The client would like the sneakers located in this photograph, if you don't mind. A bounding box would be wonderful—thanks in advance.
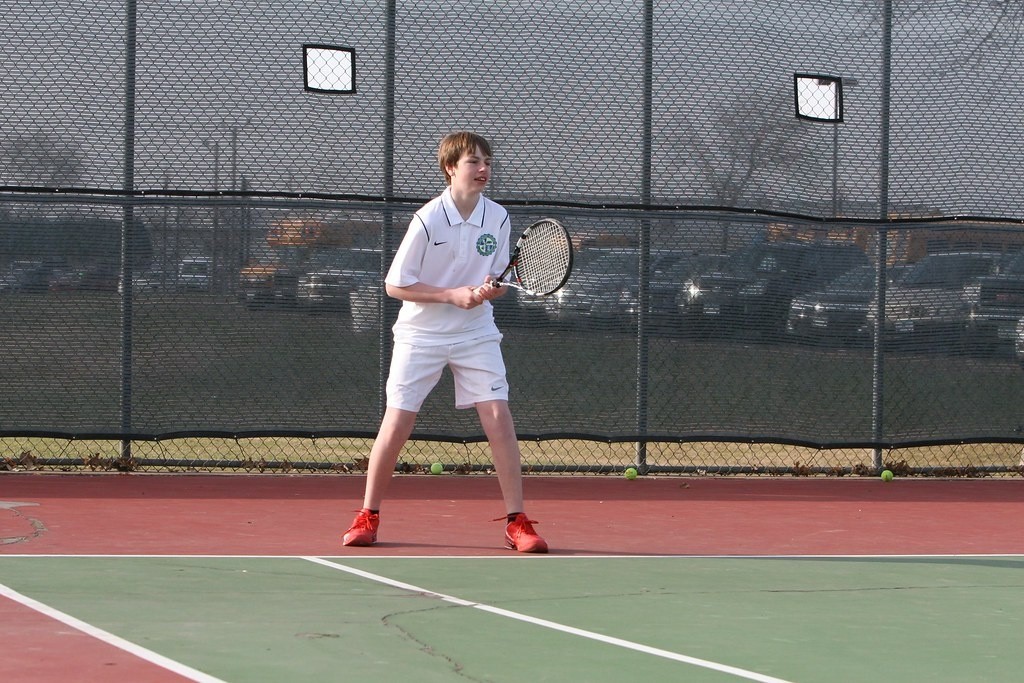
[488,513,548,553]
[340,509,380,546]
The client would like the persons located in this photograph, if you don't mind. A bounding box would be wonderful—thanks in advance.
[342,132,548,552]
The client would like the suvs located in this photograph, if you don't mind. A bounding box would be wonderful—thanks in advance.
[679,241,868,333]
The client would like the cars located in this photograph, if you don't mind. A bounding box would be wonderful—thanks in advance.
[508,248,703,318]
[968,252,1024,357]
[3,250,384,312]
[866,250,1002,343]
[787,262,910,336]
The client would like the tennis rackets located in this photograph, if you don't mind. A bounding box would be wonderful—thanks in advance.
[472,217,575,298]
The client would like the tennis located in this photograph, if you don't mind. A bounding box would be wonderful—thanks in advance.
[626,468,637,480]
[431,463,442,474]
[881,470,893,482]
